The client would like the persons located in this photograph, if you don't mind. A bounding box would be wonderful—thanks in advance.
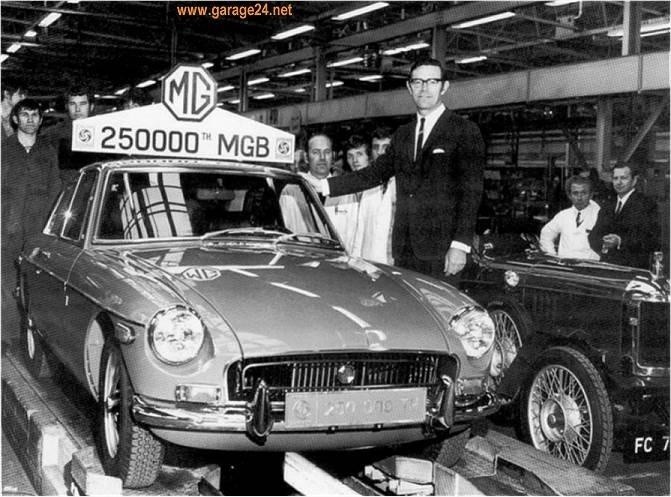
[350,126,400,268]
[39,84,106,193]
[297,55,487,295]
[338,132,373,202]
[1,69,30,144]
[1,96,62,357]
[538,171,604,262]
[588,160,663,270]
[280,131,358,255]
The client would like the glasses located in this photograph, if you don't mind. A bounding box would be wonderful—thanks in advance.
[410,78,441,88]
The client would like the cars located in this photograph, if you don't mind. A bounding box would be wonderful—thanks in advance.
[445,209,669,481]
[14,161,498,489]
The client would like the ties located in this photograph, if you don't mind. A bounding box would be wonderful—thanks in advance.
[576,212,581,225]
[615,201,622,215]
[416,119,424,158]
